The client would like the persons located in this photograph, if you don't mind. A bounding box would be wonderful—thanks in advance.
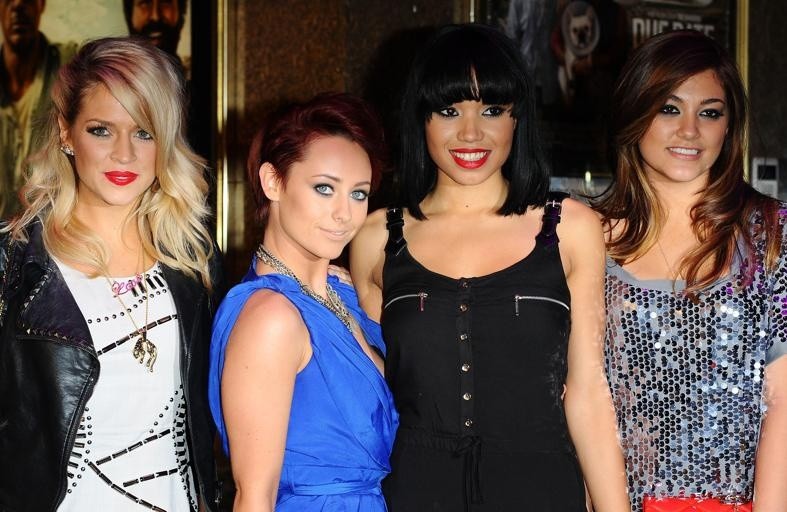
[0,35,236,512]
[207,105,399,512]
[588,29,787,512]
[349,22,632,512]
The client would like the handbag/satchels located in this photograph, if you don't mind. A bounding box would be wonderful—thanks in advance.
[642,490,753,512]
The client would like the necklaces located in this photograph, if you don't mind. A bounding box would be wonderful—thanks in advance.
[255,242,357,341]
[104,243,160,374]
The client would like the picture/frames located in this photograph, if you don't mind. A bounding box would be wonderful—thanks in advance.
[452,1,754,202]
[1,1,250,261]
